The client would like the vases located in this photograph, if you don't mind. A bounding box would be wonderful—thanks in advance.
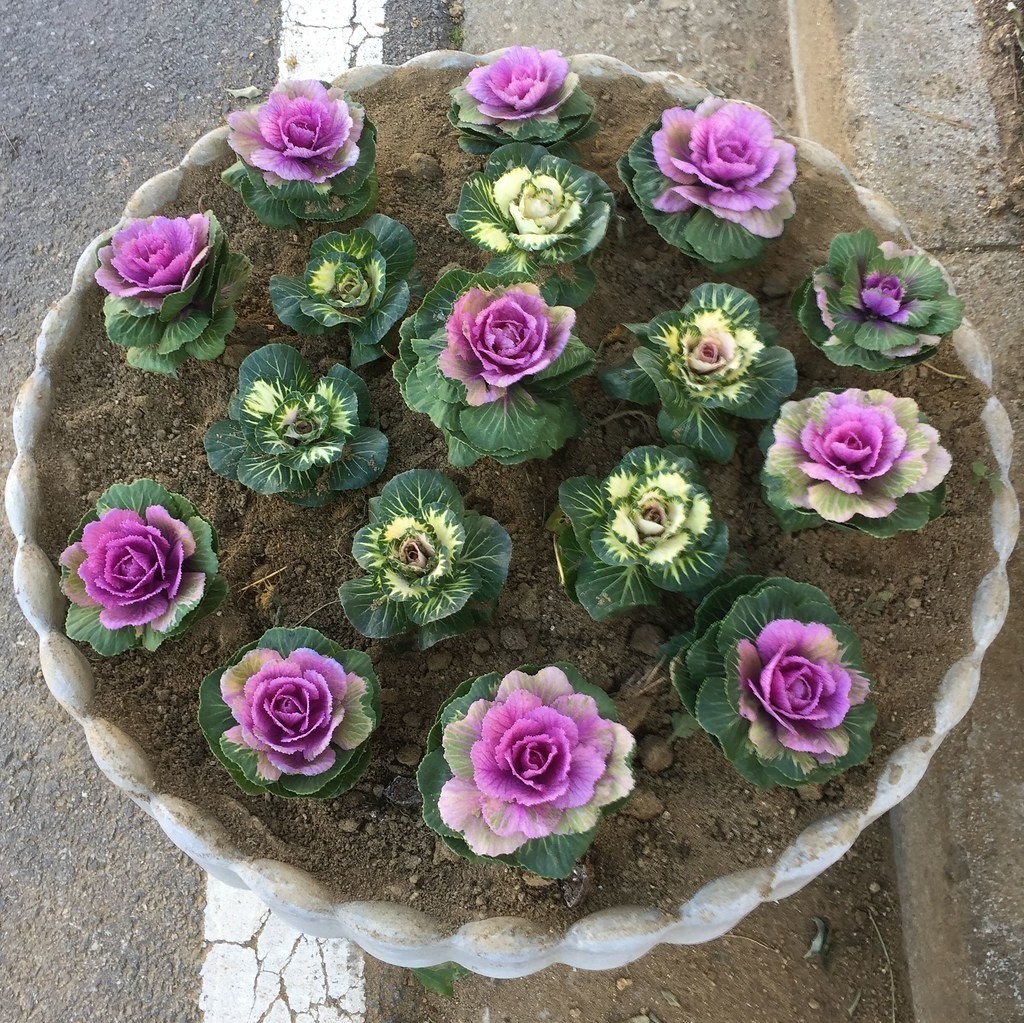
[4,49,1020,982]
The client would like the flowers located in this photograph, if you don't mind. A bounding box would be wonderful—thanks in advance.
[391,270,596,470]
[615,95,800,270]
[755,382,956,540]
[268,213,419,368]
[337,468,514,652]
[203,342,390,508]
[55,476,224,658]
[549,444,733,620]
[220,79,380,230]
[416,657,640,881]
[93,210,251,376]
[596,281,799,466]
[659,572,882,789]
[196,624,386,801]
[797,226,965,370]
[450,46,602,164]
[446,141,617,308]
